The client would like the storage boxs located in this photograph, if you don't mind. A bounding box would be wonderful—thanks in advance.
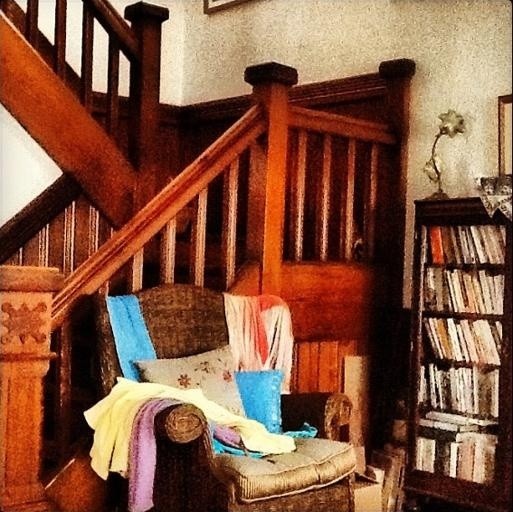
[351,471,383,512]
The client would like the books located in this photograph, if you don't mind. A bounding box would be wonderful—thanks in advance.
[412,223,507,487]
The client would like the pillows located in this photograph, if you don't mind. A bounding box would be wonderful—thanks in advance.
[132,344,247,418]
[235,364,286,432]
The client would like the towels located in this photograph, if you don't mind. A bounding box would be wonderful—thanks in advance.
[104,292,157,383]
[82,376,317,512]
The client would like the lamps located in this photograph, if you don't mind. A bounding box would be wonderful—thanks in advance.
[423,110,466,198]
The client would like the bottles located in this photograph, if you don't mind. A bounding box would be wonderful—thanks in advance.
[391,400,409,446]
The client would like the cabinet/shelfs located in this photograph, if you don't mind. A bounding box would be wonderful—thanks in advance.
[400,196,512,512]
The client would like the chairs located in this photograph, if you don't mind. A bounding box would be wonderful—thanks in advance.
[94,283,356,512]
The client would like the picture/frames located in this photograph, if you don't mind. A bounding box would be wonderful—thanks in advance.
[497,94,512,179]
[202,0,250,13]
